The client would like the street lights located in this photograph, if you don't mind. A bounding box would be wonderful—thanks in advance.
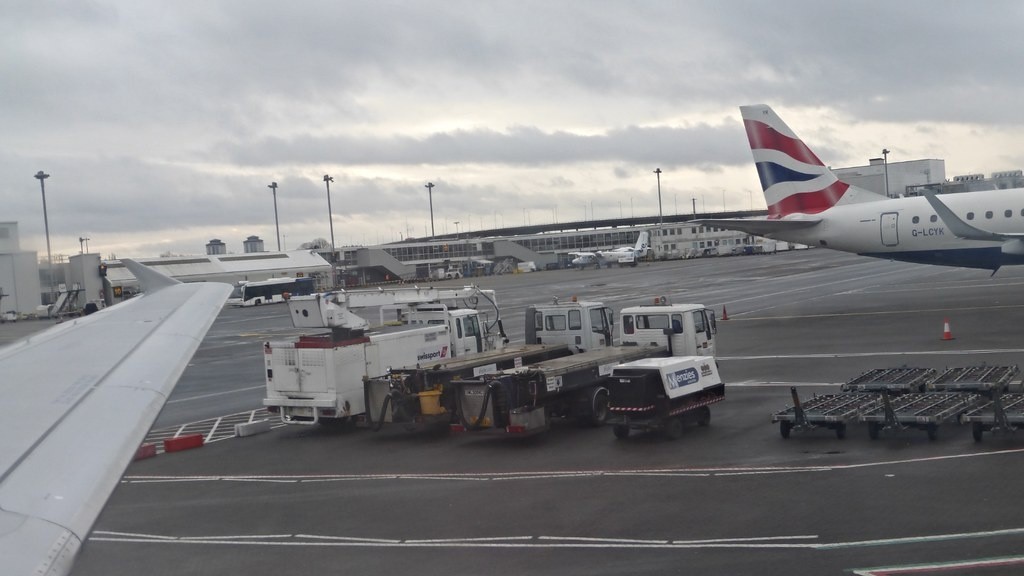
[881,148,890,197]
[454,221,460,238]
[653,168,664,242]
[268,181,283,252]
[323,174,335,263]
[34,170,57,304]
[425,181,435,239]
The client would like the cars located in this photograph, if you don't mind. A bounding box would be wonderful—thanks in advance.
[654,247,718,261]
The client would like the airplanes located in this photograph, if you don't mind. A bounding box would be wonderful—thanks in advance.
[686,104,1024,278]
[568,231,649,271]
[0,256,235,576]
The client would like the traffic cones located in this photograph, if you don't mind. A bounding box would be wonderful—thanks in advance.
[720,304,730,321]
[939,317,956,341]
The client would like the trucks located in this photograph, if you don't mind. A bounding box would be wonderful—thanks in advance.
[362,294,614,433]
[260,281,509,428]
[732,243,763,256]
[443,259,494,280]
[448,294,718,437]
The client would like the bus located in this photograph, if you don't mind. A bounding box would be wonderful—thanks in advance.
[240,276,318,307]
